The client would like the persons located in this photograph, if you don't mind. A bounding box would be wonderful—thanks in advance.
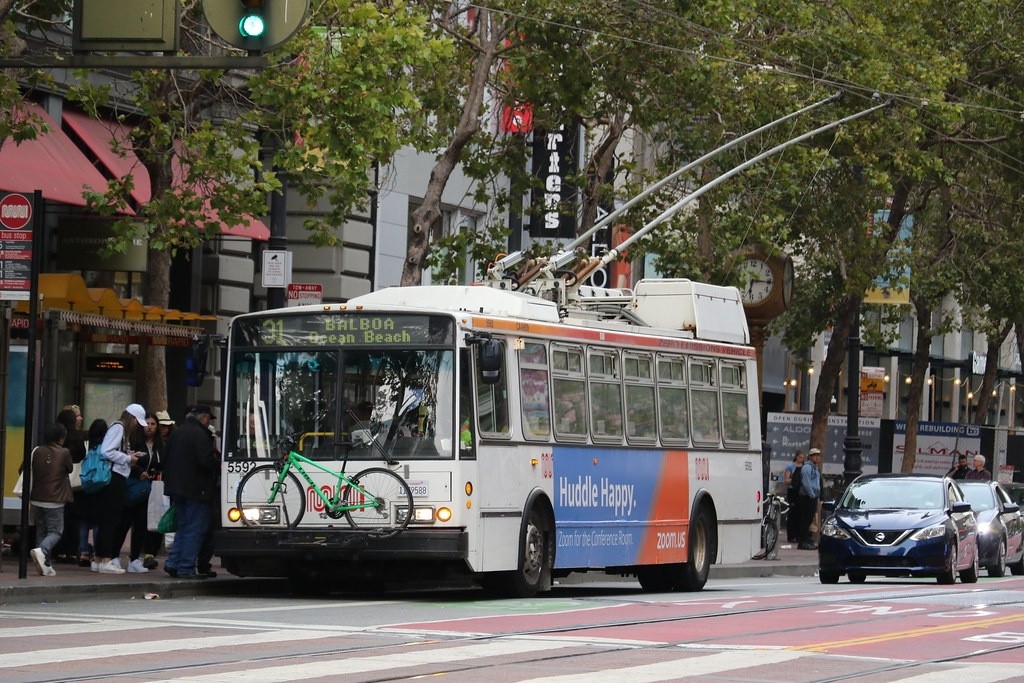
[964,455,991,481]
[797,448,820,550]
[785,450,805,544]
[945,455,971,479]
[416,399,508,454]
[18,404,221,579]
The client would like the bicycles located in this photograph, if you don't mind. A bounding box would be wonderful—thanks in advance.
[236,427,413,542]
[751,493,779,560]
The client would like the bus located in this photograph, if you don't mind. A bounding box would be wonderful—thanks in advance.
[220,89,894,598]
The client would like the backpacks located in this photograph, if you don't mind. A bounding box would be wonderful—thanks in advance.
[78,423,124,490]
[790,463,813,490]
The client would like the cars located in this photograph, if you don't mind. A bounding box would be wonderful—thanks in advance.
[817,472,980,585]
[954,480,1024,577]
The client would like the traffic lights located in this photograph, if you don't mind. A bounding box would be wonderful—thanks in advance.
[238,0,266,38]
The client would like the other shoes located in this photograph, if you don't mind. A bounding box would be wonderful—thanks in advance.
[78,552,94,567]
[788,539,797,543]
[199,568,217,577]
[127,559,149,573]
[44,565,56,576]
[29,547,46,576]
[112,558,121,568]
[143,556,159,569]
[90,561,118,571]
[97,562,126,574]
[177,571,207,579]
[163,566,180,579]
[797,543,816,551]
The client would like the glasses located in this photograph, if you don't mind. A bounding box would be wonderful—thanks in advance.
[69,405,77,411]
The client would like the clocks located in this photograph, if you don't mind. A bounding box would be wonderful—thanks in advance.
[784,261,792,303]
[734,258,773,302]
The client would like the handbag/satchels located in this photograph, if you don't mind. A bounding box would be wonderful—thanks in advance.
[146,475,170,531]
[12,446,38,499]
[156,502,176,533]
[68,441,89,488]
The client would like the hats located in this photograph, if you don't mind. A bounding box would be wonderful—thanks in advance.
[125,404,148,427]
[190,405,217,420]
[806,449,821,459]
[207,425,218,438]
[155,410,176,426]
